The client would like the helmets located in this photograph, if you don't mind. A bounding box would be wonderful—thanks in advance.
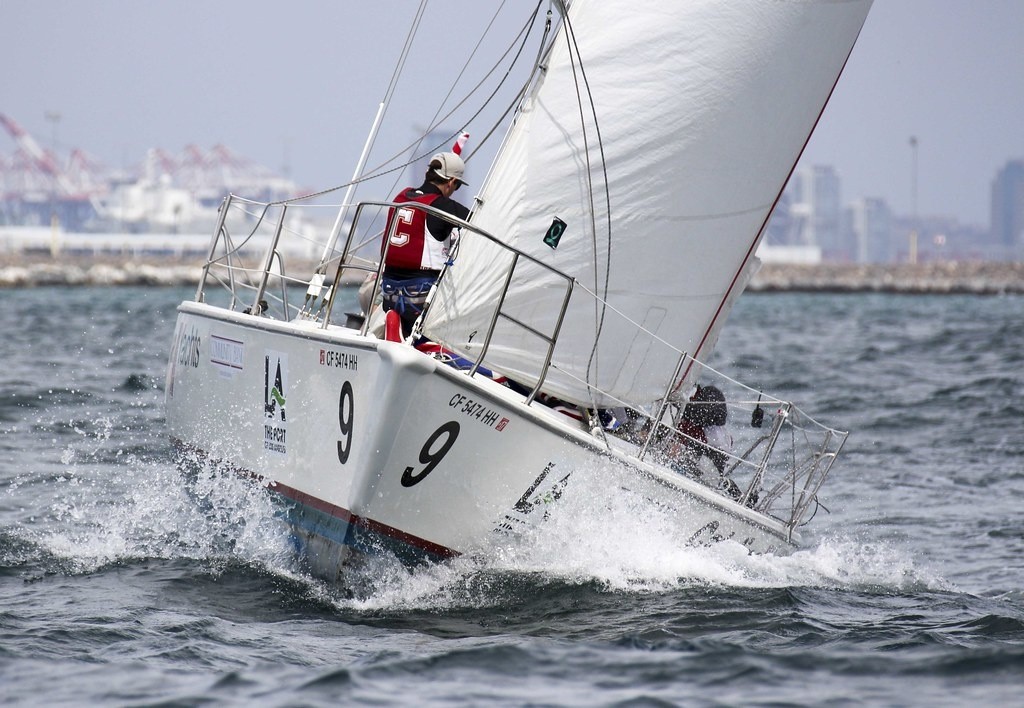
[684,384,727,427]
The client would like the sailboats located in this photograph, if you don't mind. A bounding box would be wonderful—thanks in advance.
[160,0,875,599]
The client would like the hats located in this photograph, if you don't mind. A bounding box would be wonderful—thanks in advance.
[429,152,470,186]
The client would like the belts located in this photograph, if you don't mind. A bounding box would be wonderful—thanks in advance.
[384,295,426,304]
[386,284,429,294]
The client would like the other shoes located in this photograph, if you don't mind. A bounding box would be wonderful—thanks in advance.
[384,309,405,343]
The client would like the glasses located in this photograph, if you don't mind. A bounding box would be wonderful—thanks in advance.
[455,180,462,190]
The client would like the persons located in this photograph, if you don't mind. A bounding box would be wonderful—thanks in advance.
[381,153,473,344]
